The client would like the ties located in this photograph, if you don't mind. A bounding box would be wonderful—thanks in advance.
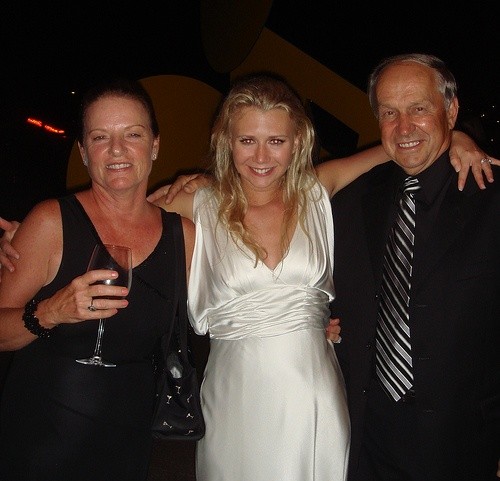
[375,176,422,402]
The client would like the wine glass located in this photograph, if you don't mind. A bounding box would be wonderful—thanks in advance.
[76,244,132,368]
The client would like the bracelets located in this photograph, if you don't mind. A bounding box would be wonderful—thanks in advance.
[23,296,64,343]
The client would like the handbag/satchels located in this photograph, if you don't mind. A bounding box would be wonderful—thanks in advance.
[151,212,210,441]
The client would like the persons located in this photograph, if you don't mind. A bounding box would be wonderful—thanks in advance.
[0,74,341,481]
[0,69,500,481]
[146,53,500,481]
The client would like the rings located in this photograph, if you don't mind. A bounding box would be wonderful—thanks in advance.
[480,158,492,164]
[88,299,97,311]
